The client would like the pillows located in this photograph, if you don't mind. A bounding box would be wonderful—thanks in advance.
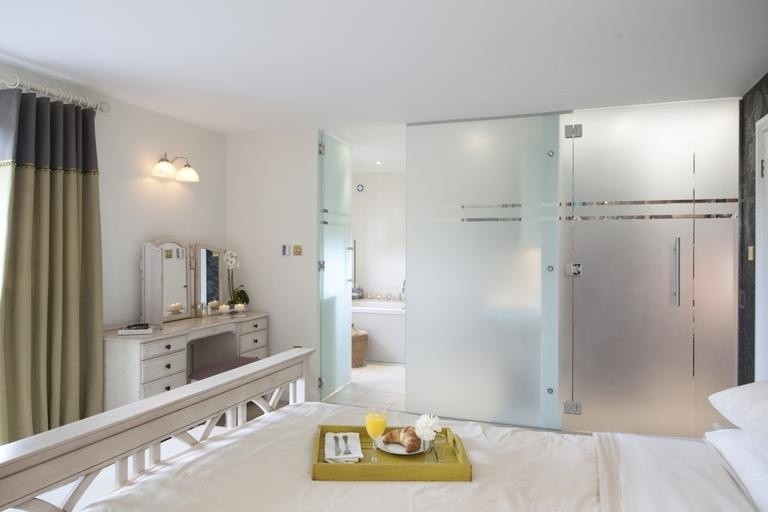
[701,366,766,509]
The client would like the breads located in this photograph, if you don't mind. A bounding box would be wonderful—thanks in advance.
[382,427,421,453]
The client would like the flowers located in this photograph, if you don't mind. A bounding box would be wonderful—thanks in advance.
[222,246,251,303]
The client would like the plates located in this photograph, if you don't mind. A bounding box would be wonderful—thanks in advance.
[375,432,429,455]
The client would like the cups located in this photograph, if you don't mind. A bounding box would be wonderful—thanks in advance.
[433,433,446,463]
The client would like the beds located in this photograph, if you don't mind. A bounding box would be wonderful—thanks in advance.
[0,345,767,511]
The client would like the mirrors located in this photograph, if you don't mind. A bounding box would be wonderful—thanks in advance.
[140,236,220,324]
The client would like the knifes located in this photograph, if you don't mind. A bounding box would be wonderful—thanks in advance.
[334,436,341,456]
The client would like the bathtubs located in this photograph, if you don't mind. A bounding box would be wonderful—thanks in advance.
[351,297,405,364]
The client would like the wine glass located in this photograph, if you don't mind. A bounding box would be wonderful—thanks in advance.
[365,408,386,463]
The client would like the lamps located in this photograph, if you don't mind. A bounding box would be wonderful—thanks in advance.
[151,151,199,182]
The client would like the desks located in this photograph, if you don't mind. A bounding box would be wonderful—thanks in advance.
[102,311,267,410]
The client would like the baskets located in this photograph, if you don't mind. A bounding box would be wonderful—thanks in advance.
[352,329,367,369]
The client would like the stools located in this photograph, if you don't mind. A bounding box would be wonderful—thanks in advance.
[189,356,260,382]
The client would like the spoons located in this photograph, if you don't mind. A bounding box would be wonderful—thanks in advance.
[343,436,351,454]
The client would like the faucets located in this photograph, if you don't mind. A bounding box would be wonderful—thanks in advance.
[400,277,405,295]
